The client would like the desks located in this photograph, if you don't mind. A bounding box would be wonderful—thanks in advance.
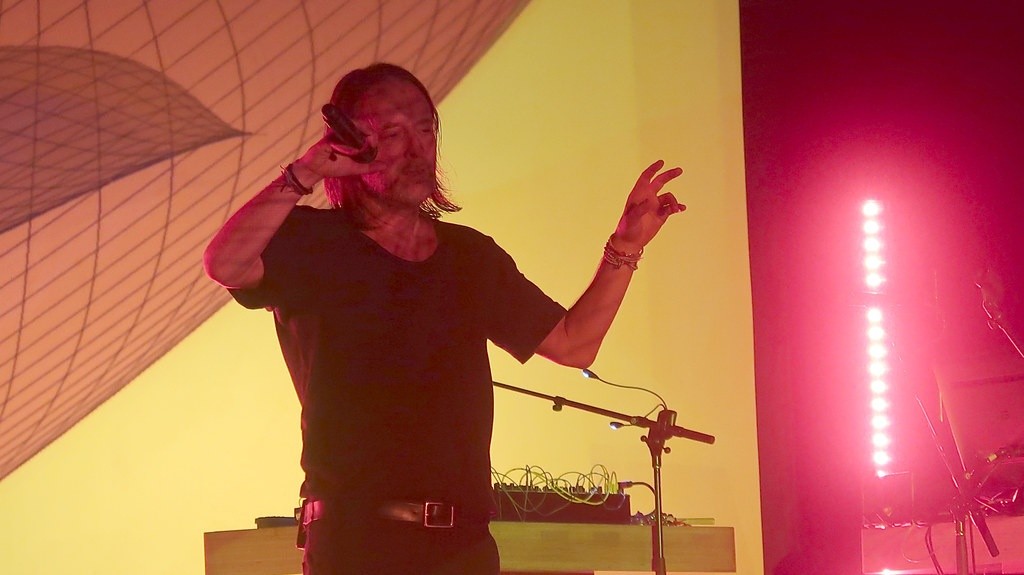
[204,520,734,575]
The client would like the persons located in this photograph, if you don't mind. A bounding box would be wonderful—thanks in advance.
[202,62,686,575]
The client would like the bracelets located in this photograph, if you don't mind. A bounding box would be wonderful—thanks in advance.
[604,234,643,270]
[280,162,313,196]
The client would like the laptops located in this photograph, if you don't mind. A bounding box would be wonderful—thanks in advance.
[862,472,914,528]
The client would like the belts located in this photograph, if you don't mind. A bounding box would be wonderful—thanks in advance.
[302,498,501,532]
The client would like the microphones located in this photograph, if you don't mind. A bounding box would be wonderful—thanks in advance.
[972,268,1002,321]
[984,438,1024,465]
[321,103,378,164]
